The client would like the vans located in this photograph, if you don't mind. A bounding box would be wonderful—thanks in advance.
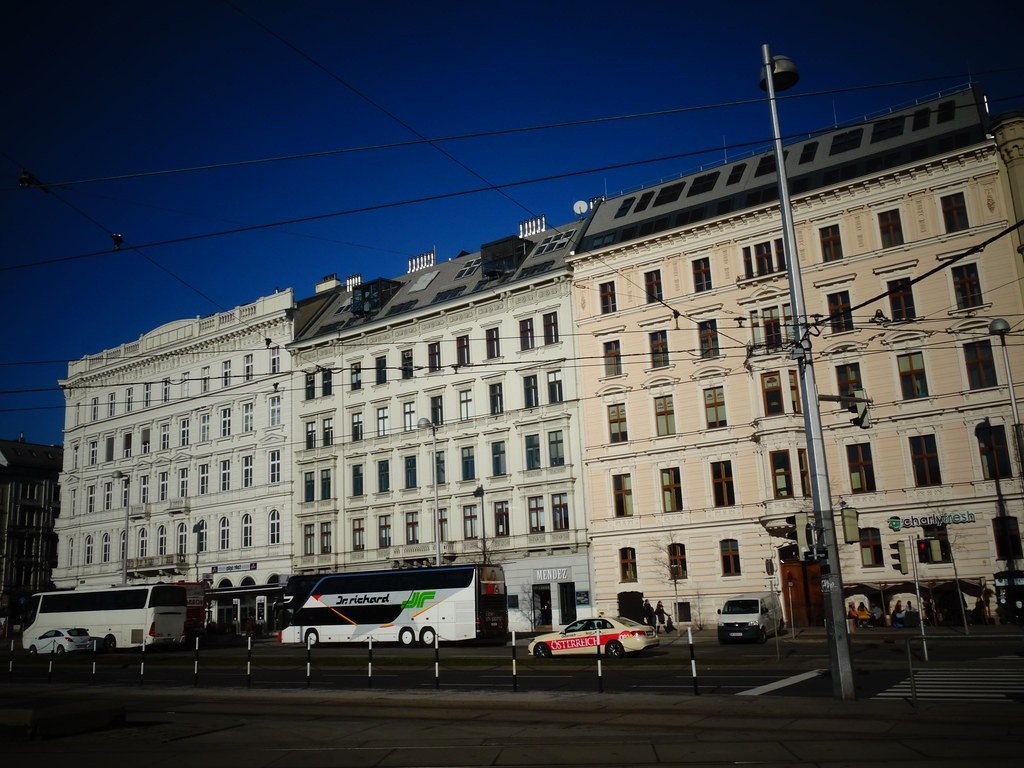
[717,592,786,645]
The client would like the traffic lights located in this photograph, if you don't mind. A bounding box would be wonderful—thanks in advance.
[785,513,811,563]
[847,391,871,429]
[889,540,909,575]
[916,539,930,563]
[940,538,951,561]
[840,509,862,545]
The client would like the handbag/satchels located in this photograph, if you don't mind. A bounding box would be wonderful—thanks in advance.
[655,609,661,616]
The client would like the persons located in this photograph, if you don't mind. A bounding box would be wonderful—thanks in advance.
[848,602,883,627]
[644,599,671,634]
[486,570,499,594]
[892,600,913,626]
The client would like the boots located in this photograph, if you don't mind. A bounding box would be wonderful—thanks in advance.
[663,626,668,633]
[657,626,662,634]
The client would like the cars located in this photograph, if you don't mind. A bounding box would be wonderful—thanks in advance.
[527,616,659,658]
[29,627,94,657]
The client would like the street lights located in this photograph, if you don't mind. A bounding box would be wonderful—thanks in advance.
[416,417,441,565]
[112,471,130,585]
[986,318,1024,474]
[756,43,854,699]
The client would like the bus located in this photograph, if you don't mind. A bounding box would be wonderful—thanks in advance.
[21,582,188,654]
[278,560,509,649]
[157,580,207,630]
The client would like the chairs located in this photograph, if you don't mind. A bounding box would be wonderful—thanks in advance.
[591,622,602,629]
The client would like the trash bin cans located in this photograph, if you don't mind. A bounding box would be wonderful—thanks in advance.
[886,614,891,626]
[848,619,857,634]
